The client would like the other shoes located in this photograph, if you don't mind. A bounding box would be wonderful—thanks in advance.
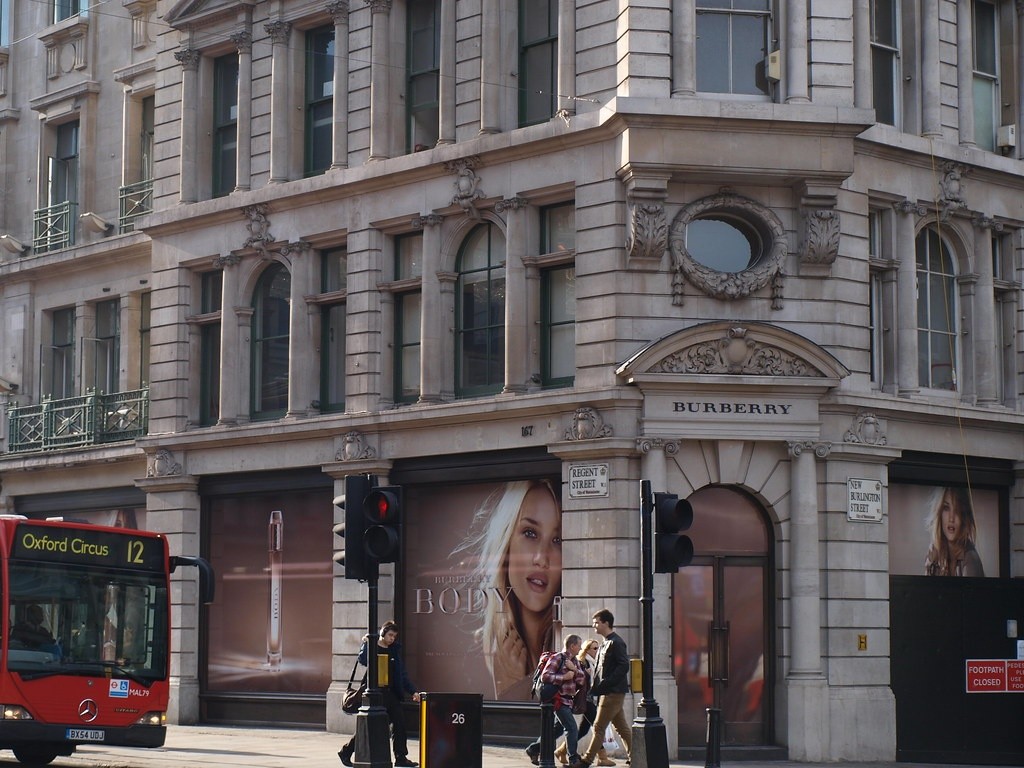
[338,750,353,767]
[563,761,589,768]
[569,758,587,768]
[525,748,539,765]
[395,757,419,767]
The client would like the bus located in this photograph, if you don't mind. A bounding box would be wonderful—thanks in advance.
[0,513,217,768]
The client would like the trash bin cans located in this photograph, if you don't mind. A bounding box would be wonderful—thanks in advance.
[419,691,483,768]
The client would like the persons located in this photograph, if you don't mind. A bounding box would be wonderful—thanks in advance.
[14,605,56,649]
[924,487,986,577]
[480,479,562,700]
[553,639,616,766]
[526,634,589,768]
[563,609,631,768]
[338,621,420,767]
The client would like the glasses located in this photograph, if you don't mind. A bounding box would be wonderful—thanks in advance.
[589,647,598,650]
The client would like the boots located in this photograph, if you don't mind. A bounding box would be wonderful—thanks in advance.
[597,749,616,766]
[554,741,568,764]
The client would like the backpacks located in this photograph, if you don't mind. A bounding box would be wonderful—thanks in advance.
[532,651,579,699]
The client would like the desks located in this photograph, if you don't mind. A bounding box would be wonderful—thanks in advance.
[107,409,138,427]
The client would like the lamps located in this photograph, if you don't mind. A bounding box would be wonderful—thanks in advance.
[0,234,31,254]
[79,212,114,233]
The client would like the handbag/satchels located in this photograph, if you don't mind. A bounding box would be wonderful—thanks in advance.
[590,722,619,750]
[531,674,559,704]
[571,683,586,715]
[341,683,367,714]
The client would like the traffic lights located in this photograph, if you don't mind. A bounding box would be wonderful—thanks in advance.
[653,491,694,574]
[360,486,402,563]
[332,473,380,581]
